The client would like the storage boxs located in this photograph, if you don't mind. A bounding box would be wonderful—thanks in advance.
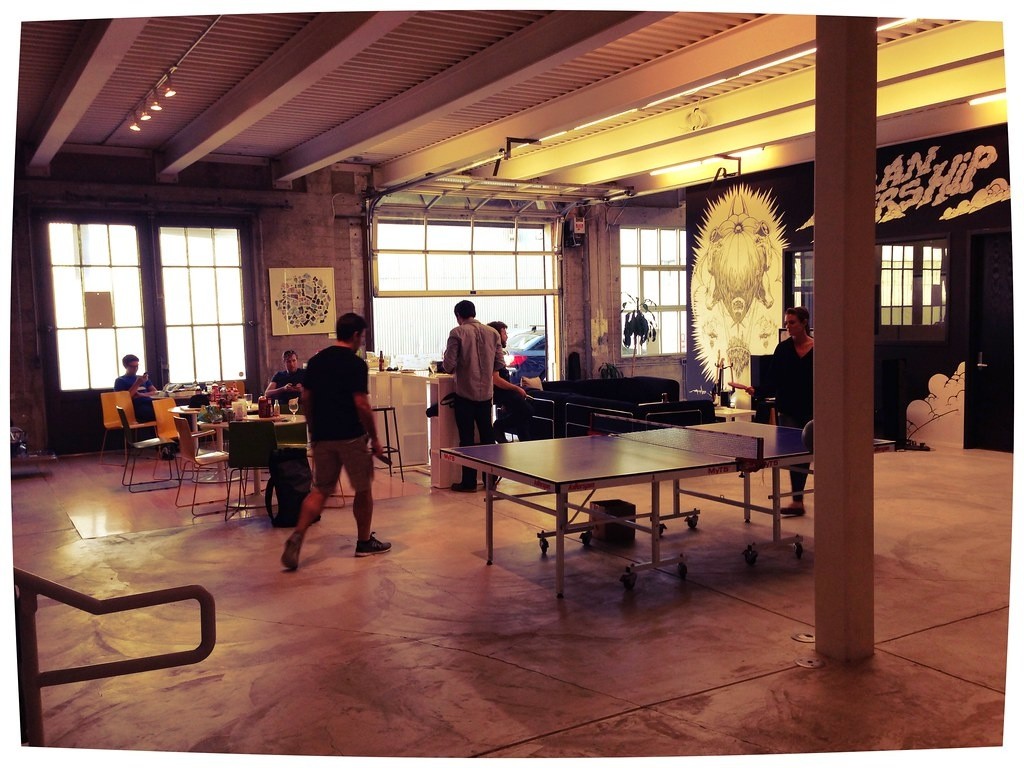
[589,499,636,541]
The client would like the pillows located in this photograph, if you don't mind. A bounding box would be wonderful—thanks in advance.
[520,376,543,390]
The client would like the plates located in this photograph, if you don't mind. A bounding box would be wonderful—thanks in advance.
[399,369,415,373]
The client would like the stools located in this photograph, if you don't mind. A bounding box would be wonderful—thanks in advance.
[372,404,406,483]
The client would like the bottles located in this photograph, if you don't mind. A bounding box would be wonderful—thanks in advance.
[196,381,247,423]
[258,392,280,418]
[379,351,384,371]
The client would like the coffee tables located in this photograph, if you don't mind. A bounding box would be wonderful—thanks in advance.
[715,405,757,422]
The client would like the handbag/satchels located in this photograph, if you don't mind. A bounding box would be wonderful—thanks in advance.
[265,448,322,527]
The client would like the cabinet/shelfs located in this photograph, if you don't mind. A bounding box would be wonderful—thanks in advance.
[429,381,441,488]
[396,378,429,468]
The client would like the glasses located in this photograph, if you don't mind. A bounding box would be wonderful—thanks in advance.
[127,365,138,369]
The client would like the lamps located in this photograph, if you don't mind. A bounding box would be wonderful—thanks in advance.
[129,73,177,132]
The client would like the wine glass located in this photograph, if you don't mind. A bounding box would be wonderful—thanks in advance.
[429,359,437,377]
[288,399,298,419]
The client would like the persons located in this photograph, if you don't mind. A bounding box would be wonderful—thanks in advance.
[265,350,306,412]
[114,354,175,459]
[444,301,505,492]
[486,321,531,443]
[282,312,392,569]
[745,307,814,514]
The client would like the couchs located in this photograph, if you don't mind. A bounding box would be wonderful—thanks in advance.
[496,376,715,441]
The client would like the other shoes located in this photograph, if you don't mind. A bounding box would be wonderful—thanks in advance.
[781,507,805,515]
[484,482,496,491]
[451,482,476,493]
[162,452,174,460]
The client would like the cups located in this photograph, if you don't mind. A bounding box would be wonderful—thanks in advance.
[243,394,252,410]
[231,402,244,422]
[238,398,247,416]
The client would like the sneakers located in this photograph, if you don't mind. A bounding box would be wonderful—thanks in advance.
[355,531,392,555]
[282,532,303,570]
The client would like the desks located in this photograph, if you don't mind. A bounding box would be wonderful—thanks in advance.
[439,413,897,602]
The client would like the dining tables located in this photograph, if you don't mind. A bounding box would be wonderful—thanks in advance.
[167,403,260,483]
[144,388,214,460]
[195,414,310,509]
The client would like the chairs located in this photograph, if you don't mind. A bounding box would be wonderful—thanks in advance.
[173,415,235,517]
[115,405,182,494]
[97,390,158,468]
[225,420,283,522]
[217,380,246,397]
[152,396,196,481]
